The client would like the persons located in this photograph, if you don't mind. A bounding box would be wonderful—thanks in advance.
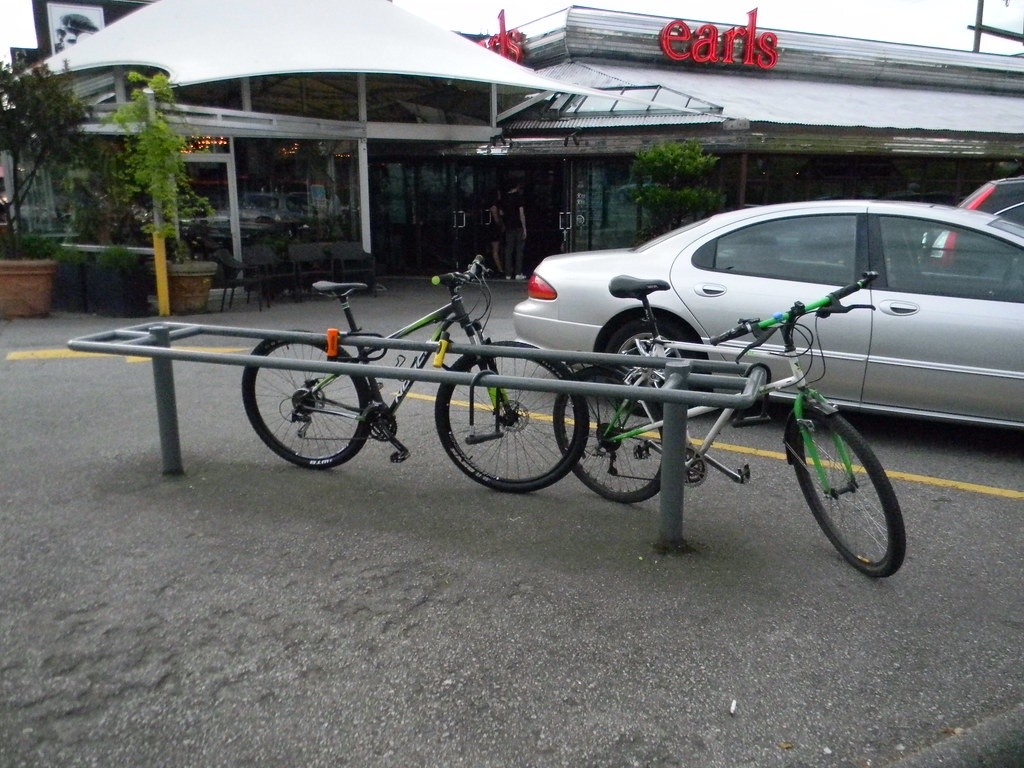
[483,188,505,278]
[498,176,527,279]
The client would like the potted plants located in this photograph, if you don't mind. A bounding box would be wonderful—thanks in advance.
[98,70,217,315]
[0,50,137,319]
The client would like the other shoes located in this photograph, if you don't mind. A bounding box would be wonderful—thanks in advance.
[505,276,512,280]
[515,273,527,281]
[498,271,504,276]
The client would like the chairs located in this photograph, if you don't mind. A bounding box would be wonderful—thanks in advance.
[216,248,271,311]
[242,240,378,302]
[889,234,914,286]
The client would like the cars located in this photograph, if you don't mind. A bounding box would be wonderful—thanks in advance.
[344,237,346,240]
[512,199,1024,431]
[239,180,347,240]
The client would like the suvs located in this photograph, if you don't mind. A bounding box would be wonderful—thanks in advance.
[921,177,1024,263]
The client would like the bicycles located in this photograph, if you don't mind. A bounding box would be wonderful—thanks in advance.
[552,272,910,578]
[239,254,590,493]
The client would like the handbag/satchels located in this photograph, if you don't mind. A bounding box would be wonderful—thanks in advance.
[515,238,534,268]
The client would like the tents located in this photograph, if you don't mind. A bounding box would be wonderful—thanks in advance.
[0,0,737,123]
